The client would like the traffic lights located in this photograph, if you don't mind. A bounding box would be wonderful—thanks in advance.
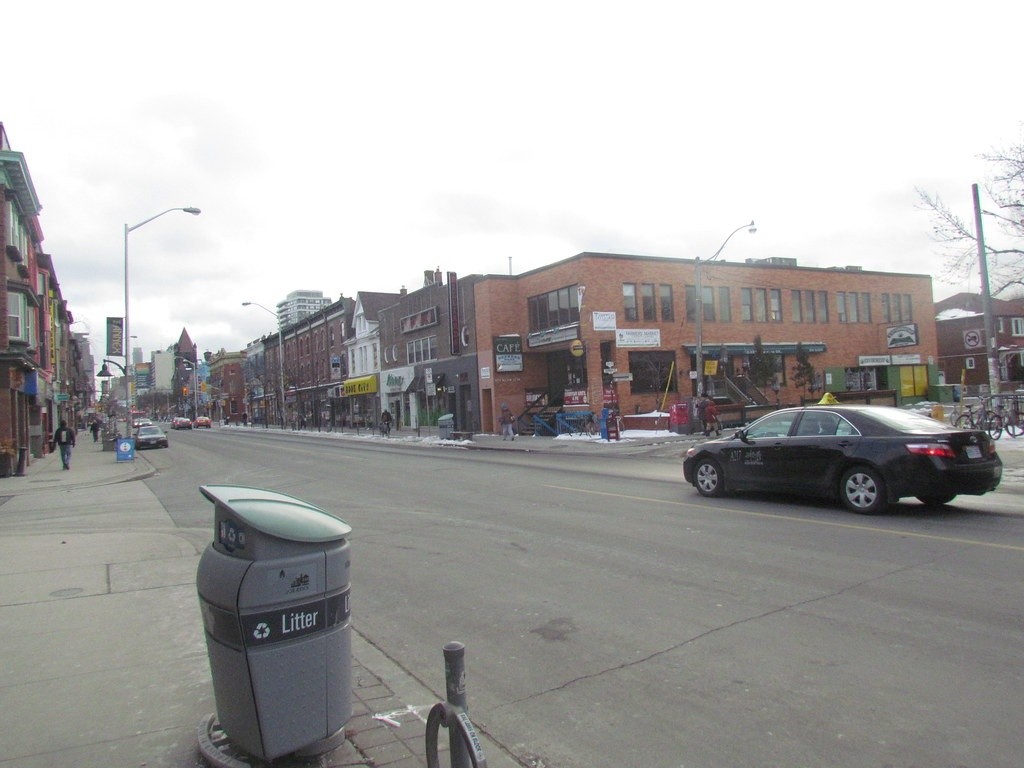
[184,388,188,396]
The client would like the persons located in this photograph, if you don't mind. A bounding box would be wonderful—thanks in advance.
[381,409,391,436]
[54,420,75,470]
[695,392,722,436]
[242,413,247,425]
[90,420,99,442]
[498,403,516,441]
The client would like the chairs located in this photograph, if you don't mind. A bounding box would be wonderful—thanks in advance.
[803,418,836,435]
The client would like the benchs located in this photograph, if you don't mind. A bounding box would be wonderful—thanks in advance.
[450,432,474,441]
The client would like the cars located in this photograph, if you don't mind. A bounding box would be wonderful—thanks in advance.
[132,426,169,450]
[194,417,211,428]
[134,419,152,428]
[683,405,1003,515]
[170,418,192,430]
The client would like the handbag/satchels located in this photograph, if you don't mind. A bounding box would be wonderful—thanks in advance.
[510,411,515,422]
[711,415,717,422]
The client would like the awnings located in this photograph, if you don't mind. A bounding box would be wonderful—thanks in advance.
[344,375,377,396]
[686,344,827,356]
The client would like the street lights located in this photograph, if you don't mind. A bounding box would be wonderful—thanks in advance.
[694,220,757,396]
[242,302,286,430]
[183,359,198,417]
[94,207,201,438]
[108,336,138,390]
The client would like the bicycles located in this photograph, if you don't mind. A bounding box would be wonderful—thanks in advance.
[988,394,1024,440]
[951,396,999,437]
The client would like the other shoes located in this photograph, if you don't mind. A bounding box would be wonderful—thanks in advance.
[706,430,710,436]
[716,431,721,436]
[702,432,706,435]
[63,463,69,470]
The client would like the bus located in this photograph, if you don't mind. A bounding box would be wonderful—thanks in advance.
[128,410,145,424]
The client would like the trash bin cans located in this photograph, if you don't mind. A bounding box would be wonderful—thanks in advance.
[438,413,454,440]
[196,483,354,768]
